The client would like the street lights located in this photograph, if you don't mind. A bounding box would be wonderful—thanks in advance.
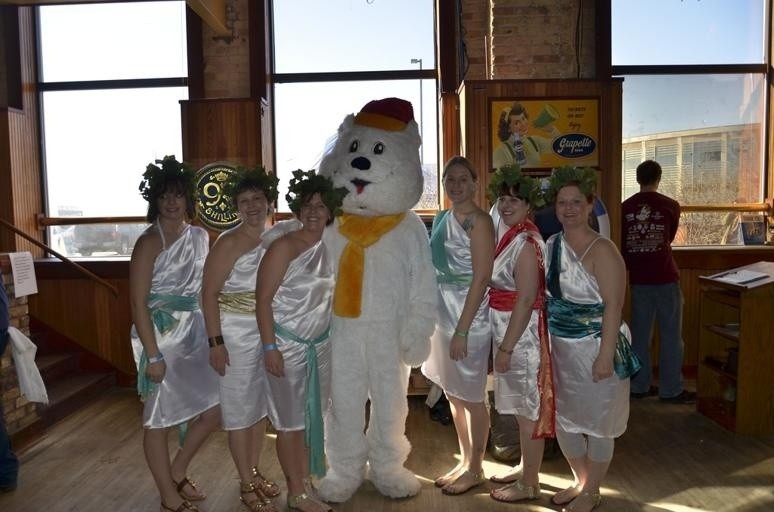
[411,58,424,166]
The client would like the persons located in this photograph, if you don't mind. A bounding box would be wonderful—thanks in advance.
[622,160,697,404]
[254,171,335,512]
[492,103,562,169]
[208,336,224,348]
[0,277,20,495]
[430,155,495,494]
[488,164,544,503]
[544,166,633,512]
[200,163,279,509]
[720,199,741,244]
[146,353,165,365]
[129,156,224,512]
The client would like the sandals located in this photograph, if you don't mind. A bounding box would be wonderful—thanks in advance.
[160,466,333,511]
[434,460,603,510]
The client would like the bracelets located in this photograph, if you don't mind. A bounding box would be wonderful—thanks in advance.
[496,345,513,355]
[262,344,278,351]
[454,328,469,337]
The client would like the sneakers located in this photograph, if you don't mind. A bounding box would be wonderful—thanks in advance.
[658,390,697,404]
[630,386,659,398]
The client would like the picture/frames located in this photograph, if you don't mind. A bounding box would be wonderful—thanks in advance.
[486,97,603,173]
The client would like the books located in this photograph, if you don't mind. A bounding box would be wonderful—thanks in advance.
[742,214,764,223]
[740,221,765,245]
[715,268,769,284]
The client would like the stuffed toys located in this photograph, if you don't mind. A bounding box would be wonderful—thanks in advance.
[260,97,437,504]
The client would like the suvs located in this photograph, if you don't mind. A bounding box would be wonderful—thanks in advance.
[74,223,145,256]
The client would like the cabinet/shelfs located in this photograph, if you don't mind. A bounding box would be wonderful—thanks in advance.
[696,262,773,447]
